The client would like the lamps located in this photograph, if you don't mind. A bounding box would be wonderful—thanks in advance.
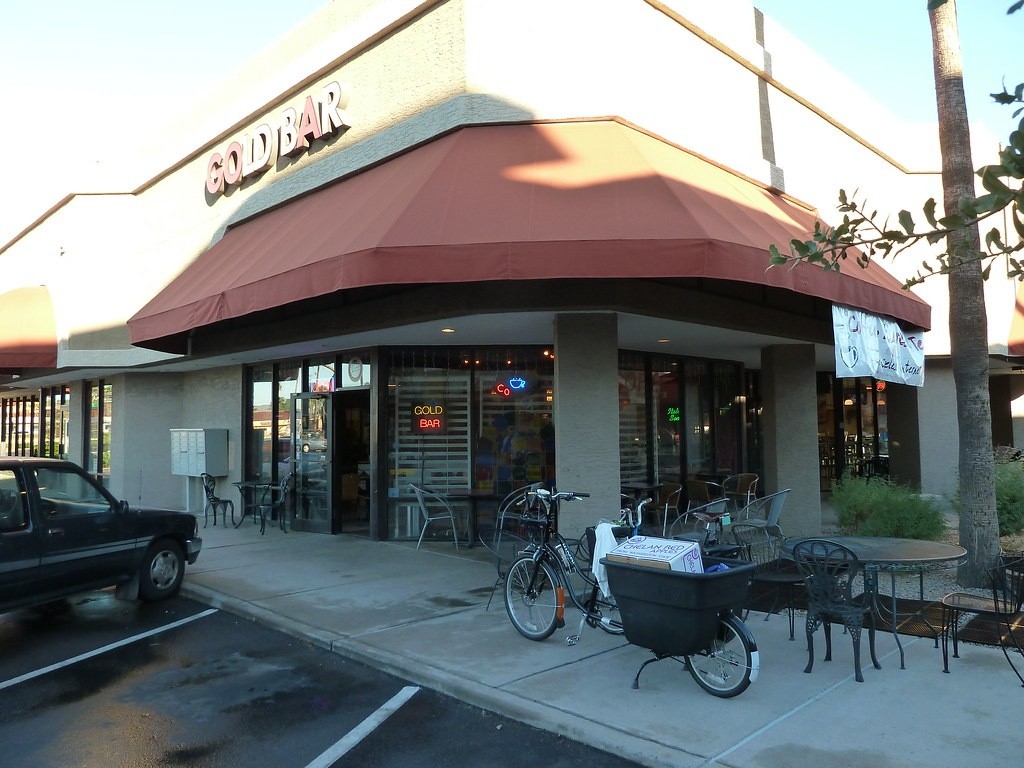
[844,379,853,405]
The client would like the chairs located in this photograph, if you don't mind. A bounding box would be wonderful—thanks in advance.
[476,528,558,611]
[258,472,296,536]
[491,481,547,553]
[649,471,1024,686]
[407,482,460,553]
[201,473,238,530]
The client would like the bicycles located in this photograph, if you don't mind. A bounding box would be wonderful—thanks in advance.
[503,488,760,698]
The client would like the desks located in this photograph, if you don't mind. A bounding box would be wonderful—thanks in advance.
[781,537,967,669]
[448,487,495,547]
[621,481,663,536]
[232,480,283,533]
[528,537,582,594]
[697,473,744,522]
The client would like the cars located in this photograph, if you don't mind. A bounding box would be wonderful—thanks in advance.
[262,436,327,489]
[0,457,202,615]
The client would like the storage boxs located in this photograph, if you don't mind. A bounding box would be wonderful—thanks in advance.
[606,534,704,573]
